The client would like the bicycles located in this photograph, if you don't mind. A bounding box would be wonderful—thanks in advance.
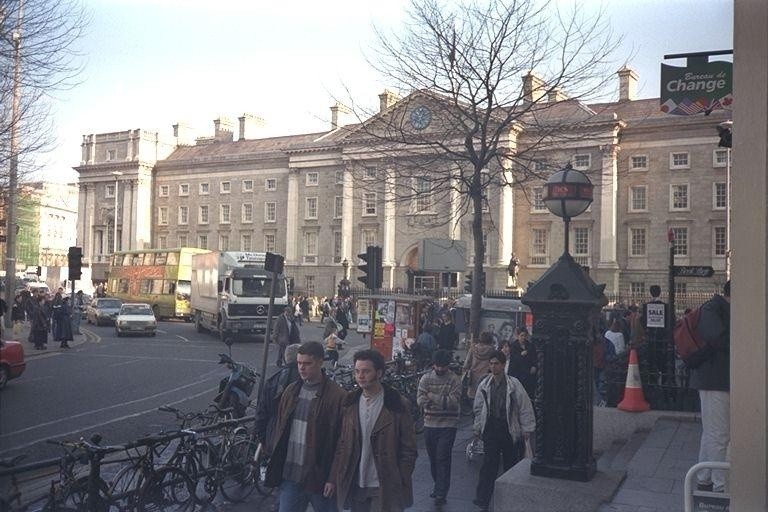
[322,350,475,434]
[0,404,275,512]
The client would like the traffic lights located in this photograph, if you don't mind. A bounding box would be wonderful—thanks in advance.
[69,247,83,280]
[464,271,485,295]
[357,246,383,289]
[37,266,41,276]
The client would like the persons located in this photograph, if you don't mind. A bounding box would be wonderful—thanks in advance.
[21,290,50,298]
[601,303,639,324]
[57,297,74,348]
[312,296,320,317]
[256,342,301,460]
[51,292,61,341]
[32,298,47,349]
[605,320,623,352]
[294,302,302,326]
[264,341,348,512]
[591,328,617,394]
[271,307,291,367]
[321,299,331,323]
[438,311,458,357]
[12,295,22,331]
[289,295,300,301]
[509,328,537,397]
[301,297,311,323]
[693,282,730,505]
[416,348,460,505]
[497,339,510,372]
[332,297,353,329]
[0,300,8,340]
[411,325,438,356]
[463,331,495,407]
[324,329,346,366]
[472,352,534,510]
[322,351,417,511]
[73,291,84,336]
[643,284,668,386]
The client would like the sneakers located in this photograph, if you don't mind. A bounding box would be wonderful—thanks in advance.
[435,495,447,507]
[473,499,489,512]
[431,488,436,497]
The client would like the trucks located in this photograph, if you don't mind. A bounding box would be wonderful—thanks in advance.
[190,251,294,342]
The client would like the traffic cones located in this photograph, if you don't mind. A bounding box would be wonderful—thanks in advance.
[614,349,652,413]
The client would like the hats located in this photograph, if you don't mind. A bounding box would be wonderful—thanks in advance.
[431,349,452,366]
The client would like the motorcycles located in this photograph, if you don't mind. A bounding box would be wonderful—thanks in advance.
[209,336,261,418]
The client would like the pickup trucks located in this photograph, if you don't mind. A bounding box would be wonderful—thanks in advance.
[114,303,157,336]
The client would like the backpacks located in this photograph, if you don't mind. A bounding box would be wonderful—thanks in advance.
[674,302,726,369]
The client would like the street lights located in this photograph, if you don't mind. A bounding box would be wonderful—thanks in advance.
[112,171,124,254]
[338,258,352,302]
[519,158,611,476]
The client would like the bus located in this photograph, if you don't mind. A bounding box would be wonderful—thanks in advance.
[104,249,213,322]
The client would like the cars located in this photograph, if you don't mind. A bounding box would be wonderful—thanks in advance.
[1,272,50,299]
[0,339,25,390]
[88,298,125,326]
